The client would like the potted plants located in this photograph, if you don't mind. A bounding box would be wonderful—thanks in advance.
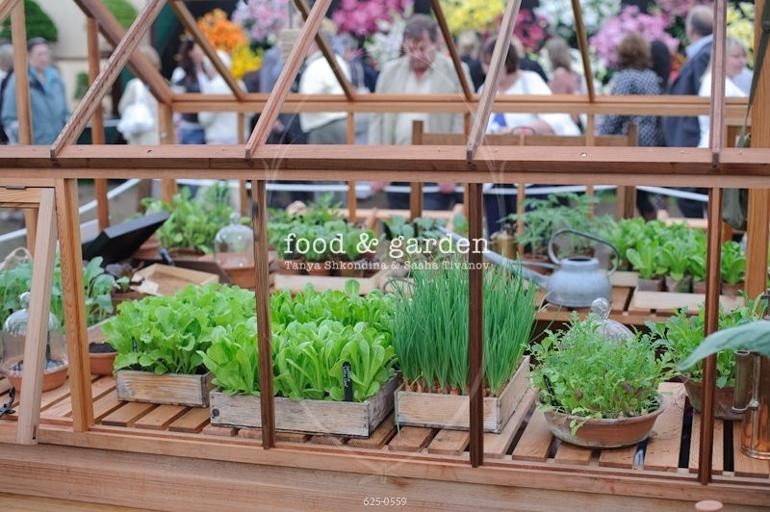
[0,178,769,462]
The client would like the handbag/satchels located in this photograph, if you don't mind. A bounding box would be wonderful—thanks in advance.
[721,131,748,229]
[117,100,155,136]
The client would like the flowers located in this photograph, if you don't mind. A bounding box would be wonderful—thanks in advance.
[178,2,757,96]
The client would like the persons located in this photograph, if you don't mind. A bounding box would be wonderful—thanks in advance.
[0,36,72,146]
[115,3,758,243]
[0,43,13,144]
